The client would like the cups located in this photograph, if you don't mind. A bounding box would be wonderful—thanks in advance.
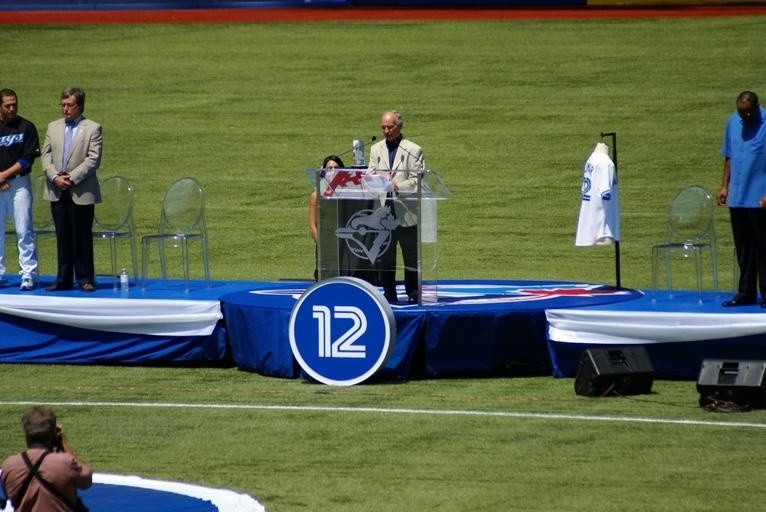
[353,140,365,165]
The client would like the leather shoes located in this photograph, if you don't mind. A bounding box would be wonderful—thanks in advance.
[44,280,75,292]
[721,291,766,309]
[78,280,95,292]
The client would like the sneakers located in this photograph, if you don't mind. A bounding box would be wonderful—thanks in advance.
[407,294,419,305]
[0,276,12,289]
[19,274,39,291]
[384,292,400,305]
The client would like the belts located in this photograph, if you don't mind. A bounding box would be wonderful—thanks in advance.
[4,170,29,182]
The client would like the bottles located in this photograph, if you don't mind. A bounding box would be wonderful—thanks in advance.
[120,267,129,292]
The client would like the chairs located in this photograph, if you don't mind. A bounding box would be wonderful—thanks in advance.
[91,175,143,291]
[651,184,721,306]
[5,176,56,290]
[733,248,761,299]
[140,175,210,294]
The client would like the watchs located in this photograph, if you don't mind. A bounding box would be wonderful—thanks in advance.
[66,176,72,182]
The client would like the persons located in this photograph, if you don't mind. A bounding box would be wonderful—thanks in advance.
[1,406,91,512]
[574,140,618,247]
[717,91,765,307]
[41,86,101,292]
[365,110,425,303]
[309,155,343,280]
[0,88,41,290]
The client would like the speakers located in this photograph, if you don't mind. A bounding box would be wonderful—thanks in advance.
[574,347,654,397]
[697,359,766,407]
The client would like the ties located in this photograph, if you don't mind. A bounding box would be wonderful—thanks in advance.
[388,144,396,171]
[61,121,76,174]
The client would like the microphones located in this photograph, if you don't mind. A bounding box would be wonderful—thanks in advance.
[334,136,376,157]
[394,140,423,164]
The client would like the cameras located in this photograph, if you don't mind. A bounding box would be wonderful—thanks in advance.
[55,428,62,449]
